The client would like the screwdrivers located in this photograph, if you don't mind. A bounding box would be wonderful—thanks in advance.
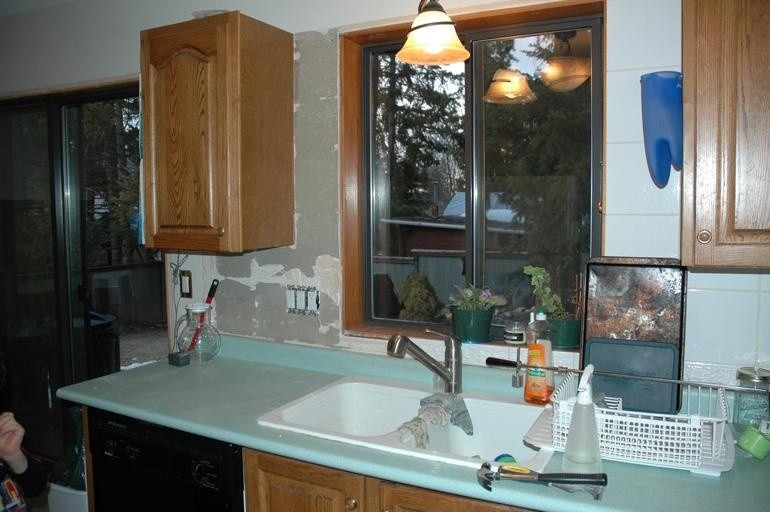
[497,464,604,498]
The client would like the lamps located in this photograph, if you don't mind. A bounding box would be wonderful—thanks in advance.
[395,1,469,67]
[482,29,591,108]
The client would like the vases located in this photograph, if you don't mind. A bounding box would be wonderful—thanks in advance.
[451,306,493,343]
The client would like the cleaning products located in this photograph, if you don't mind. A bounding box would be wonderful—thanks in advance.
[524,306,555,393]
[562,364,603,474]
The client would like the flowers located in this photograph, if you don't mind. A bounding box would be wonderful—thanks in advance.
[448,283,506,310]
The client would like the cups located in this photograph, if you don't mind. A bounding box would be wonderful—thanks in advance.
[504,319,526,346]
[737,427,770,461]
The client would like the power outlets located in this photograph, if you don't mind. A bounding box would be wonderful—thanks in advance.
[307,291,318,310]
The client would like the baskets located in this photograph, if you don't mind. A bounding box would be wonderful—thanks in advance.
[549,373,732,467]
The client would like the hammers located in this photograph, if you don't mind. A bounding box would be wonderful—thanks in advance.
[478,462,610,490]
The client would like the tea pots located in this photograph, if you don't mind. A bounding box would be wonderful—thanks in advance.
[176,303,220,360]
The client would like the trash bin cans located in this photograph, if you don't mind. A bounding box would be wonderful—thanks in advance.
[89,311,120,378]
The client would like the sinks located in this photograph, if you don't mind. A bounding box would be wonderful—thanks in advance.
[257,376,437,437]
[400,399,556,480]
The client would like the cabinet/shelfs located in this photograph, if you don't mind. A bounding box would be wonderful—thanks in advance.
[139,11,294,253]
[681,1,769,269]
[242,449,515,511]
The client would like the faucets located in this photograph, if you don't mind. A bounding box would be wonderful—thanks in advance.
[388,328,463,393]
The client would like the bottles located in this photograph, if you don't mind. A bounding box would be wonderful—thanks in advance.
[732,368,768,434]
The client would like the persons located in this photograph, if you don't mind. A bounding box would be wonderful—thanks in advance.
[0,412,47,511]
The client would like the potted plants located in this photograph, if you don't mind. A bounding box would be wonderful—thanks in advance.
[521,264,584,350]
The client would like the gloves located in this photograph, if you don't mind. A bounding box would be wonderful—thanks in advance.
[640,72,681,188]
